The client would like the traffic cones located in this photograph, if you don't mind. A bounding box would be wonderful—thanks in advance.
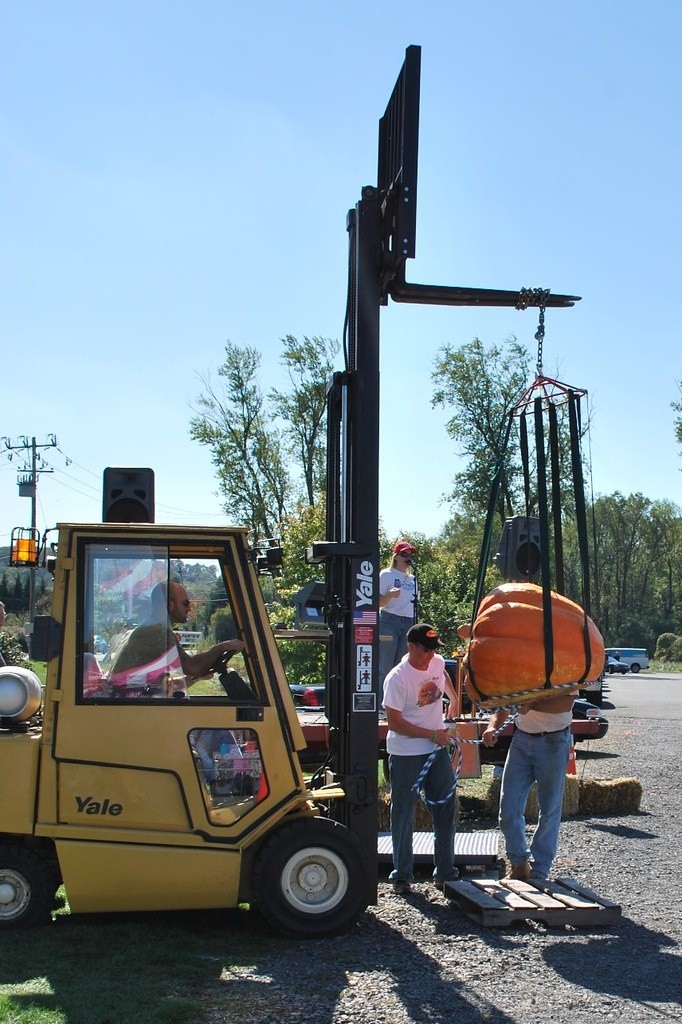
[566,735,576,775]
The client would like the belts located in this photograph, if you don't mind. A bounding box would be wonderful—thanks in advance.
[522,727,567,736]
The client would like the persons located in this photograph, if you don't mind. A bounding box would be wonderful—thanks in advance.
[194,729,238,806]
[381,623,459,893]
[378,542,418,720]
[106,581,245,698]
[482,682,579,880]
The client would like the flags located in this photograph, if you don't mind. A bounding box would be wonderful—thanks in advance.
[100,557,173,601]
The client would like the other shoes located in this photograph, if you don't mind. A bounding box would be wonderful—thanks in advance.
[433,877,445,886]
[505,862,532,880]
[392,880,410,893]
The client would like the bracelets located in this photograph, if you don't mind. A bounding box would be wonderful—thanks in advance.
[432,730,437,742]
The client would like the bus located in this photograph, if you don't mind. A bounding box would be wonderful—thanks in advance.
[604,646,651,673]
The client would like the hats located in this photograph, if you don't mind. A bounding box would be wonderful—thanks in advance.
[407,623,446,649]
[394,541,416,554]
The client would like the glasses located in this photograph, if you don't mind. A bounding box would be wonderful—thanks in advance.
[415,643,436,652]
[171,599,190,607]
[397,552,415,558]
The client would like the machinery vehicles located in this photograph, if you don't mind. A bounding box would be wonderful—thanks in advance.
[0,36,607,945]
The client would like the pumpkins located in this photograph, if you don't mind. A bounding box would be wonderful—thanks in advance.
[456,582,606,701]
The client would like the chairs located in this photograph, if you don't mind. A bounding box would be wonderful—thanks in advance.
[84,653,108,697]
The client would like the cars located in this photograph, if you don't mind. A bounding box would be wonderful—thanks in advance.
[579,673,604,707]
[291,658,610,782]
[604,656,630,674]
